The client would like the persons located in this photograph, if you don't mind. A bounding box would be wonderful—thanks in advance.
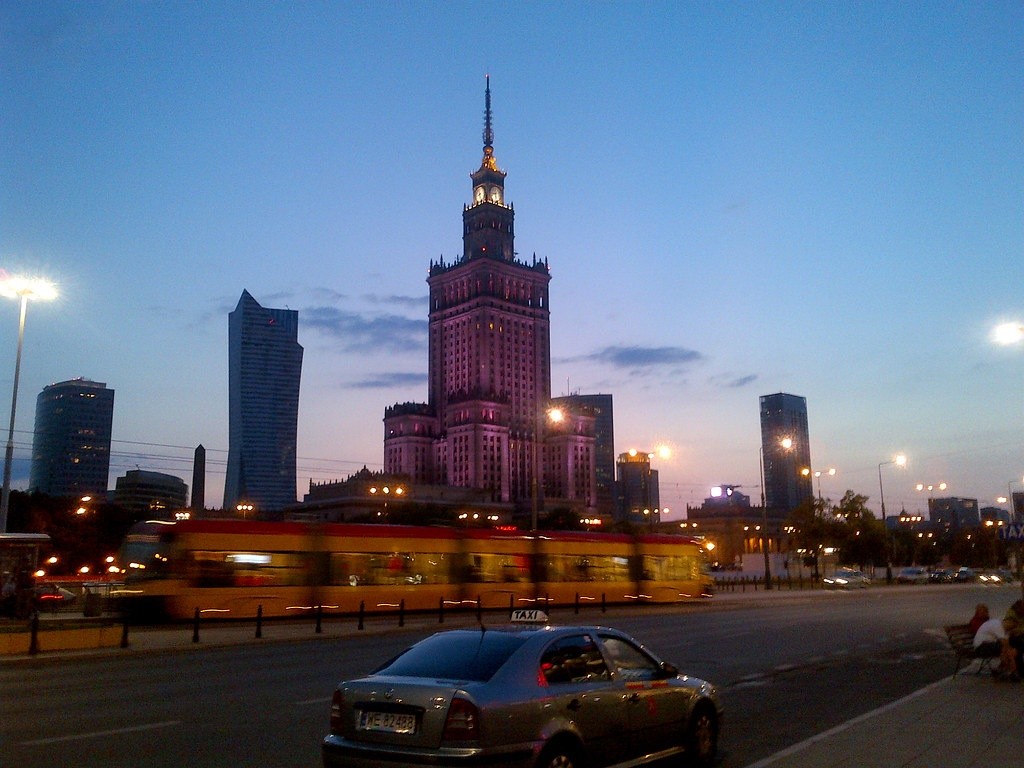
[969,598,1024,681]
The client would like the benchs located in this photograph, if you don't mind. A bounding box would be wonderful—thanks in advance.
[943,624,997,680]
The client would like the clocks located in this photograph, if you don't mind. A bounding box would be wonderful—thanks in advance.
[488,183,504,205]
[473,183,487,205]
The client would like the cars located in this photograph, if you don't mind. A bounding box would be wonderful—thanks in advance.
[823,571,870,589]
[898,567,1012,582]
[321,611,722,768]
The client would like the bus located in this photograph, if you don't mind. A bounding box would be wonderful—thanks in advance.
[126,518,715,620]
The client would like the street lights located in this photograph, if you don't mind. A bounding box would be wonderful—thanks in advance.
[815,468,836,515]
[760,438,792,590]
[1,273,57,533]
[878,456,906,584]
